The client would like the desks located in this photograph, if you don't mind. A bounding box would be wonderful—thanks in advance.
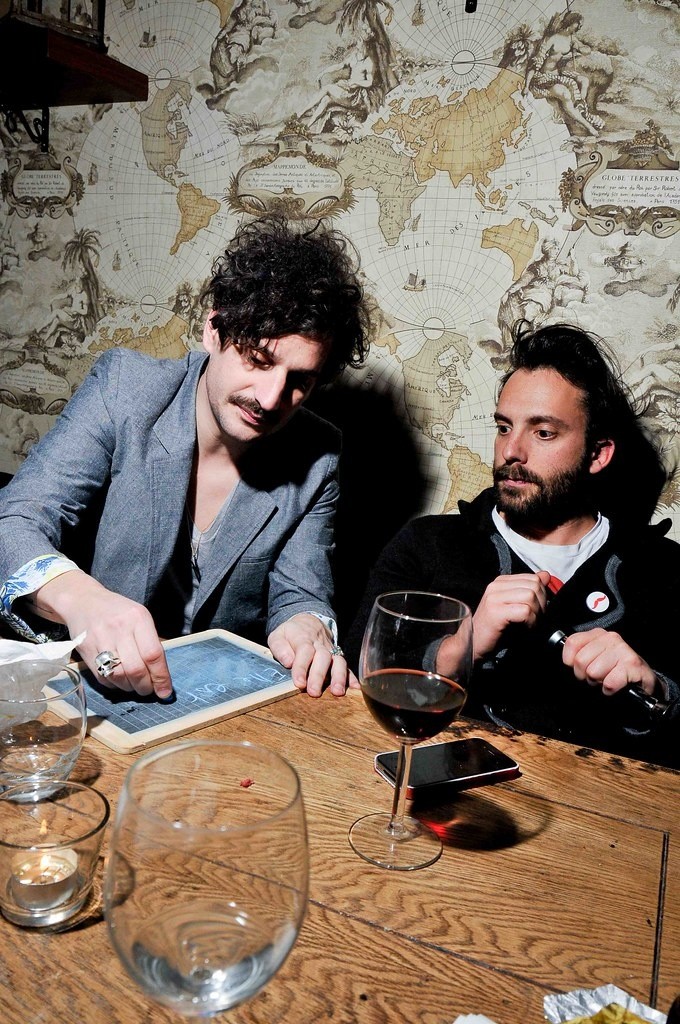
[0,636,680,1024]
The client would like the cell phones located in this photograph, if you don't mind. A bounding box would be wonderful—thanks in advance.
[374,737,519,800]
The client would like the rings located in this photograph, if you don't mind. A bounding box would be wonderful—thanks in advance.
[95,651,121,678]
[330,646,344,656]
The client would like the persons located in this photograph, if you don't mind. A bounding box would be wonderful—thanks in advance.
[349,323,679,773]
[0,214,361,696]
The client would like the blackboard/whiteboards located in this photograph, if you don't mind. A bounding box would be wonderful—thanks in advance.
[41,629,303,755]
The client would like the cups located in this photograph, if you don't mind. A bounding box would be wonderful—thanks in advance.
[0,659,88,800]
[105,736,310,1017]
[0,779,105,927]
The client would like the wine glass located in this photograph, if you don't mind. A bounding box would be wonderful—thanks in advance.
[348,587,475,871]
